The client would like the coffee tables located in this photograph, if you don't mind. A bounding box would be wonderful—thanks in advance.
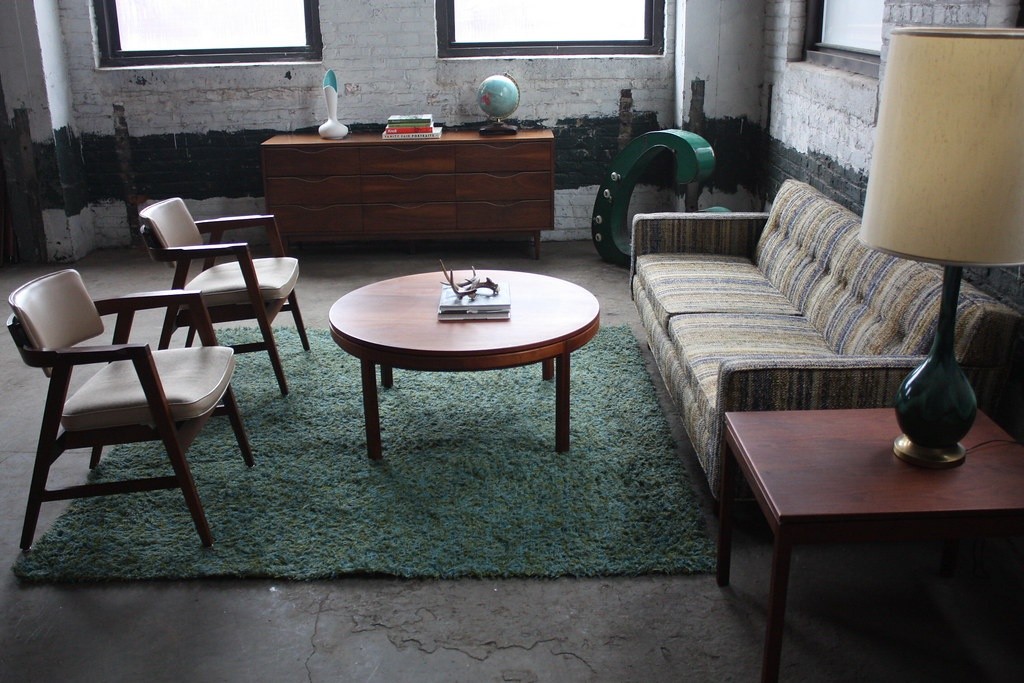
[328,268,601,457]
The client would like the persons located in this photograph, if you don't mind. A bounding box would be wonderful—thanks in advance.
[438,310,510,320]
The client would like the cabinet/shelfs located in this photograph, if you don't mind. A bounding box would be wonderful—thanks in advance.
[259,127,555,261]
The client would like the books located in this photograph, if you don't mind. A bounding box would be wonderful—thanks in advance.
[438,281,510,312]
[381,114,443,139]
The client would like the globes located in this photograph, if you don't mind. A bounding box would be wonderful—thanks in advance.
[479,71,521,137]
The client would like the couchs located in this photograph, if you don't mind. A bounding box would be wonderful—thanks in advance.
[630,179,1024,521]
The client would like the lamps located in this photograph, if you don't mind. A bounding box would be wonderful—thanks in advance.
[857,23,1024,472]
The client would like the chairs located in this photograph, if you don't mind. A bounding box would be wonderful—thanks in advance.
[137,197,311,395]
[7,269,255,550]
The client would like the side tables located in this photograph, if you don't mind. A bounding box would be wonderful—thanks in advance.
[716,405,1023,683]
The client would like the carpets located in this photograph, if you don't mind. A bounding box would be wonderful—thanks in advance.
[13,324,712,583]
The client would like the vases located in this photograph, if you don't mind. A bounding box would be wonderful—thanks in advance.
[318,69,349,141]
